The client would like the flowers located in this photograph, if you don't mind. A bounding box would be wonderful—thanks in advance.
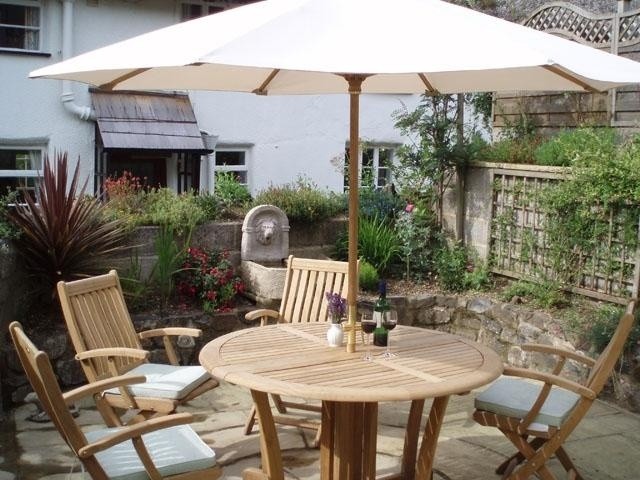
[174,246,246,316]
[323,293,346,323]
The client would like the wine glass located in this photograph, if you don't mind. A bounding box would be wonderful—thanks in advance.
[381,309,400,360]
[360,312,378,362]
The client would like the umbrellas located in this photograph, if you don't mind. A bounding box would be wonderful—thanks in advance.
[28,1,639,351]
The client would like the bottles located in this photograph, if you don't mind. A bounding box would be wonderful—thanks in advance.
[374,279,389,347]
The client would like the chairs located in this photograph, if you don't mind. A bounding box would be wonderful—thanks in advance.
[243,254,360,447]
[472,298,637,479]
[57,269,219,428]
[9,322,223,480]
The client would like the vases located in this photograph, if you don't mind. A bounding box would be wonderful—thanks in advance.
[328,323,342,348]
